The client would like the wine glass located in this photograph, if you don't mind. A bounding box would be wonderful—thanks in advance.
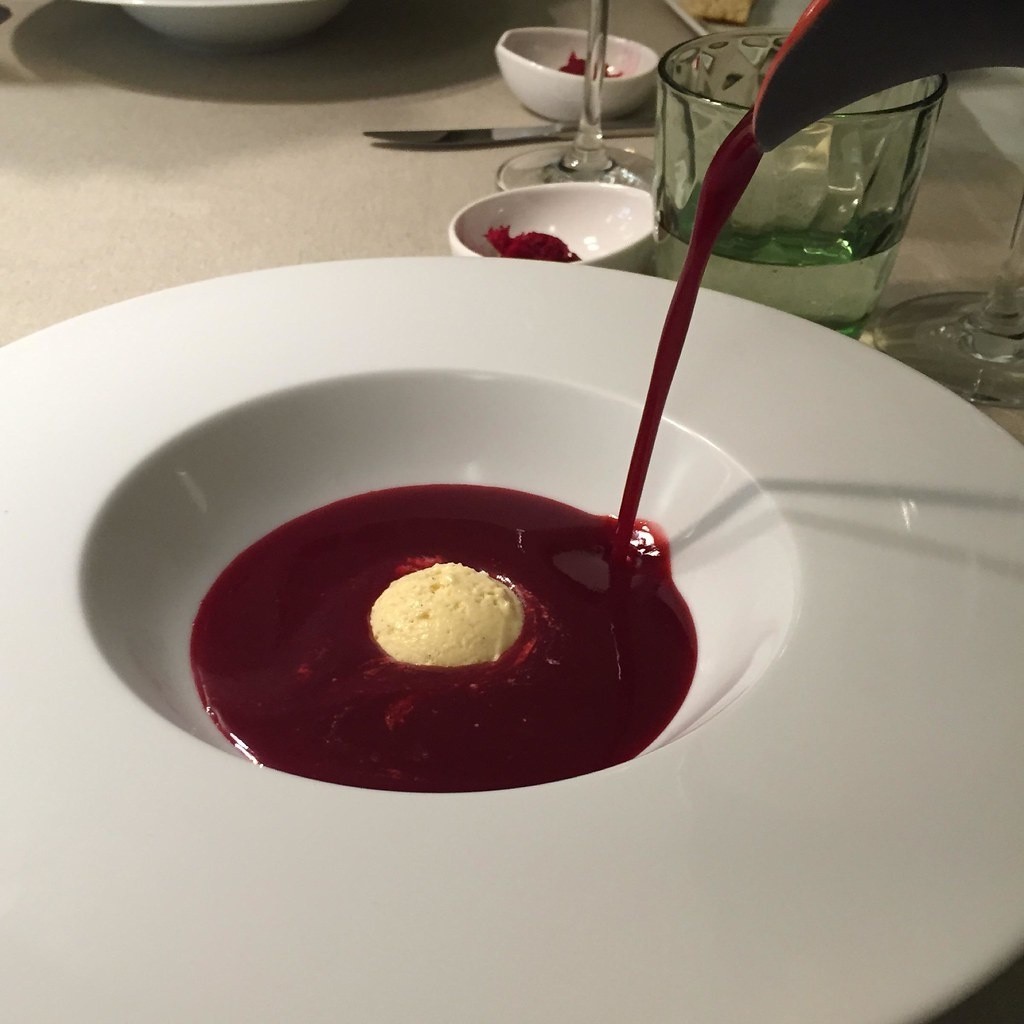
[496,0,655,191]
[871,195,1024,409]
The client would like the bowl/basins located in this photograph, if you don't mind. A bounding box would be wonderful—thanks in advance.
[81,0,350,44]
[495,27,658,122]
[449,182,657,273]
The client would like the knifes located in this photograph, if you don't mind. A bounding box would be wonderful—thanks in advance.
[362,118,652,146]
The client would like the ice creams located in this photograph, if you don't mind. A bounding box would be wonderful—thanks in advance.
[367,561,524,667]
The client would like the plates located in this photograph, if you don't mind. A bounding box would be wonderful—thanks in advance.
[663,0,812,40]
[2,254,1023,1024]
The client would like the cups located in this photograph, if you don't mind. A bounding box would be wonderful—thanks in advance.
[653,28,951,339]
